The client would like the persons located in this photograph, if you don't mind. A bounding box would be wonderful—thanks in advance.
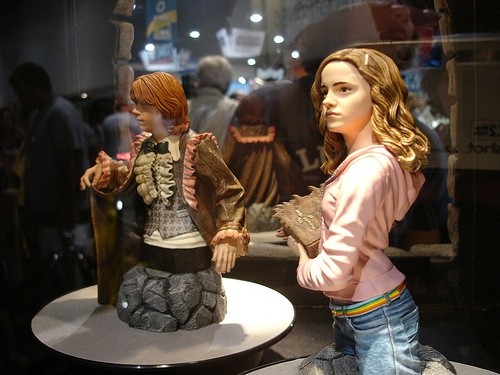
[81,72,252,274]
[0,22,450,232]
[286,48,431,375]
